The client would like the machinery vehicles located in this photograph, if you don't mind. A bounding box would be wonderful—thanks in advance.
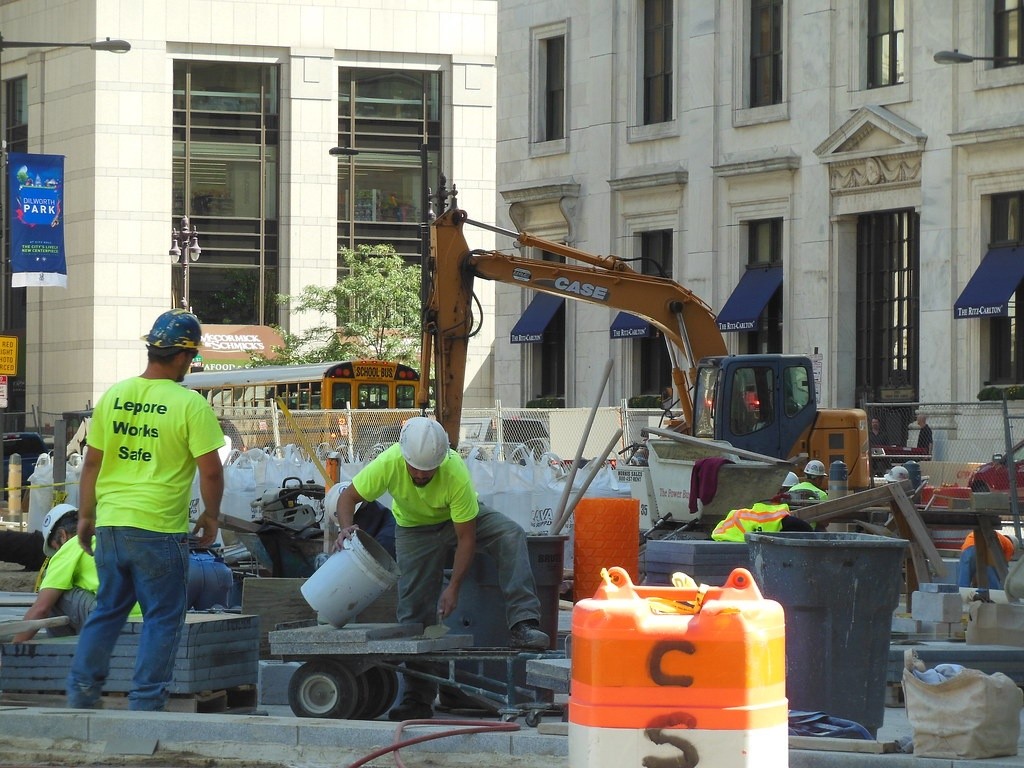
[418,183,872,491]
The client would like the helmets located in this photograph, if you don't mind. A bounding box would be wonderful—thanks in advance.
[398,416,448,470]
[781,471,799,486]
[1004,534,1019,555]
[140,307,204,347]
[884,466,909,482]
[43,504,80,557]
[804,460,827,476]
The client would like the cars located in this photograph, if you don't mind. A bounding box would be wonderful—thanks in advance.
[969,437,1024,506]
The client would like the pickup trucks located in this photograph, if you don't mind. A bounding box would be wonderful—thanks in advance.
[3,432,53,497]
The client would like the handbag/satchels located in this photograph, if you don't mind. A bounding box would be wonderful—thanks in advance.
[901,649,1022,759]
[787,710,873,740]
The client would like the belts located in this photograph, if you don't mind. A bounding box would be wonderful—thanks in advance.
[190,547,217,554]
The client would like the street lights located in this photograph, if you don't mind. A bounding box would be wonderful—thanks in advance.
[329,145,429,313]
[1,39,131,430]
[170,225,200,311]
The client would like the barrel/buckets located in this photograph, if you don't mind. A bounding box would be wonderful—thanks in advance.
[301,528,401,629]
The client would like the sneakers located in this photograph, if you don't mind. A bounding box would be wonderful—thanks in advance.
[509,621,551,649]
[388,693,433,718]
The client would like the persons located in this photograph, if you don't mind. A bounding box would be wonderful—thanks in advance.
[326,417,550,719]
[869,417,890,478]
[187,532,233,611]
[16,504,141,639]
[67,308,226,714]
[957,531,1018,591]
[711,460,829,542]
[916,414,933,462]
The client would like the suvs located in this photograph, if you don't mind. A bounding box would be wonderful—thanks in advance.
[457,418,550,461]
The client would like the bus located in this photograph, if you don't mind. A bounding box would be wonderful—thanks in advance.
[180,360,419,457]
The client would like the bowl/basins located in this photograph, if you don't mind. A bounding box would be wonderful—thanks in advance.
[923,487,971,506]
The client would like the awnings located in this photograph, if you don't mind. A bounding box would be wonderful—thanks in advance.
[610,312,649,339]
[954,246,1024,319]
[715,265,783,331]
[510,292,565,343]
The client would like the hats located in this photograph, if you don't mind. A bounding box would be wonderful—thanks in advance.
[146,344,185,356]
